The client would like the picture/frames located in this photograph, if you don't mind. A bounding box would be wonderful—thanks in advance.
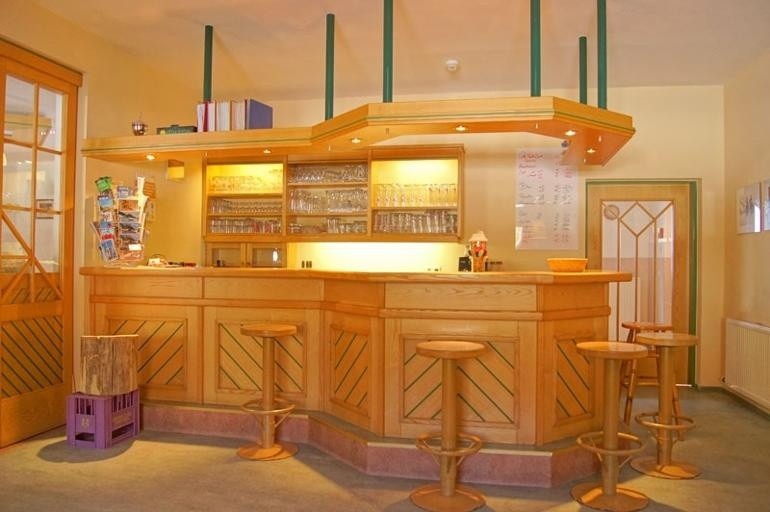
[35,198,55,220]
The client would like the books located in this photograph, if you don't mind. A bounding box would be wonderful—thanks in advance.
[196,97,273,133]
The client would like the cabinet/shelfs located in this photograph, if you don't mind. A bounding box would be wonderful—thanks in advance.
[201,143,464,243]
[205,243,299,268]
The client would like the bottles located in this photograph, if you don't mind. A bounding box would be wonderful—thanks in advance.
[466,232,488,272]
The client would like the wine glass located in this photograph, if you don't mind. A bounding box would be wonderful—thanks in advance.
[289,165,368,213]
[209,198,282,214]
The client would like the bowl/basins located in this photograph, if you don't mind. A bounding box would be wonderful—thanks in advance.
[547,258,590,272]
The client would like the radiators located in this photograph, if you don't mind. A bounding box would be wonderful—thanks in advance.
[720,317,769,414]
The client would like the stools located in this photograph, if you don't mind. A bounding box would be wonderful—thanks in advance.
[409,341,486,512]
[235,324,300,460]
[569,320,701,510]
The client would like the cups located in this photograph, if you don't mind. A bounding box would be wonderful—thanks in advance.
[289,216,367,236]
[373,183,460,232]
[210,218,280,234]
